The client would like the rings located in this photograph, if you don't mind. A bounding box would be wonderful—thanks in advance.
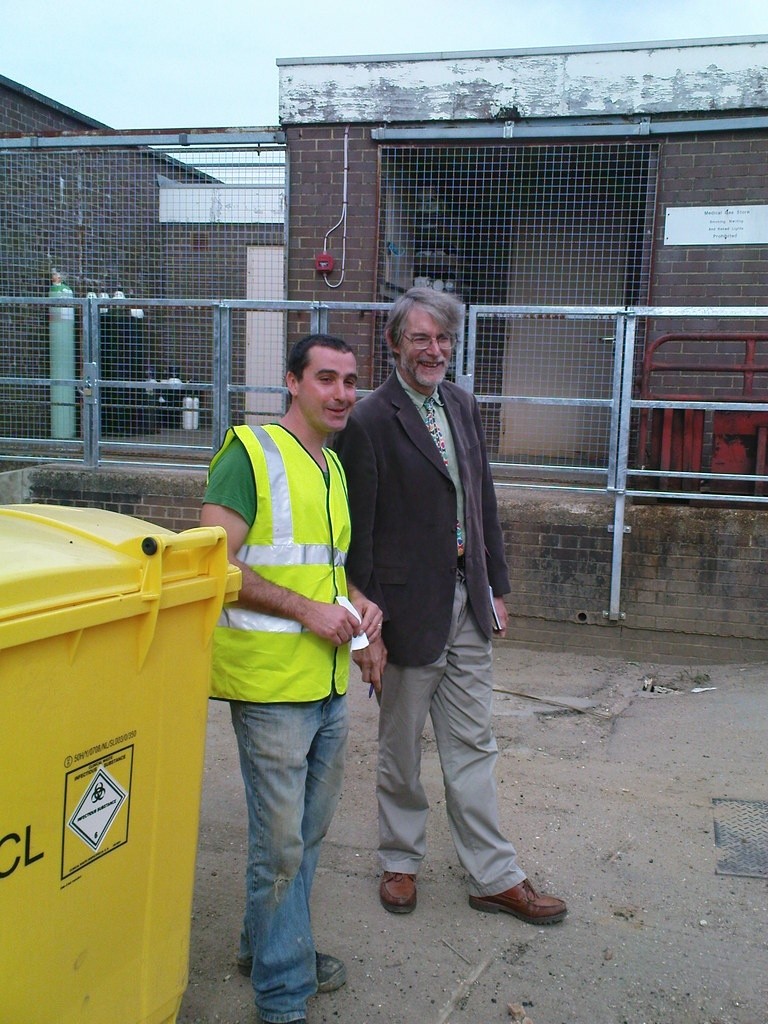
[378,624,381,627]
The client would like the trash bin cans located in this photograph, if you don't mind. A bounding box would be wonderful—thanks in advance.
[0,503,243,1022]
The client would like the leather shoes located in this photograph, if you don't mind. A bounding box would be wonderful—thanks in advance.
[468,878,567,926]
[379,870,418,914]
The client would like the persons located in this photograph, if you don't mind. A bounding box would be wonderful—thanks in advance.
[335,285,567,927]
[197,333,383,1024]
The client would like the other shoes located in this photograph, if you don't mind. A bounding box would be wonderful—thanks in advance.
[237,950,349,993]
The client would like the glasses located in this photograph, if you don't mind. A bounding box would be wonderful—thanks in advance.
[399,330,457,350]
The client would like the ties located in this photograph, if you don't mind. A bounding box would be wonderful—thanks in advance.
[422,398,464,557]
